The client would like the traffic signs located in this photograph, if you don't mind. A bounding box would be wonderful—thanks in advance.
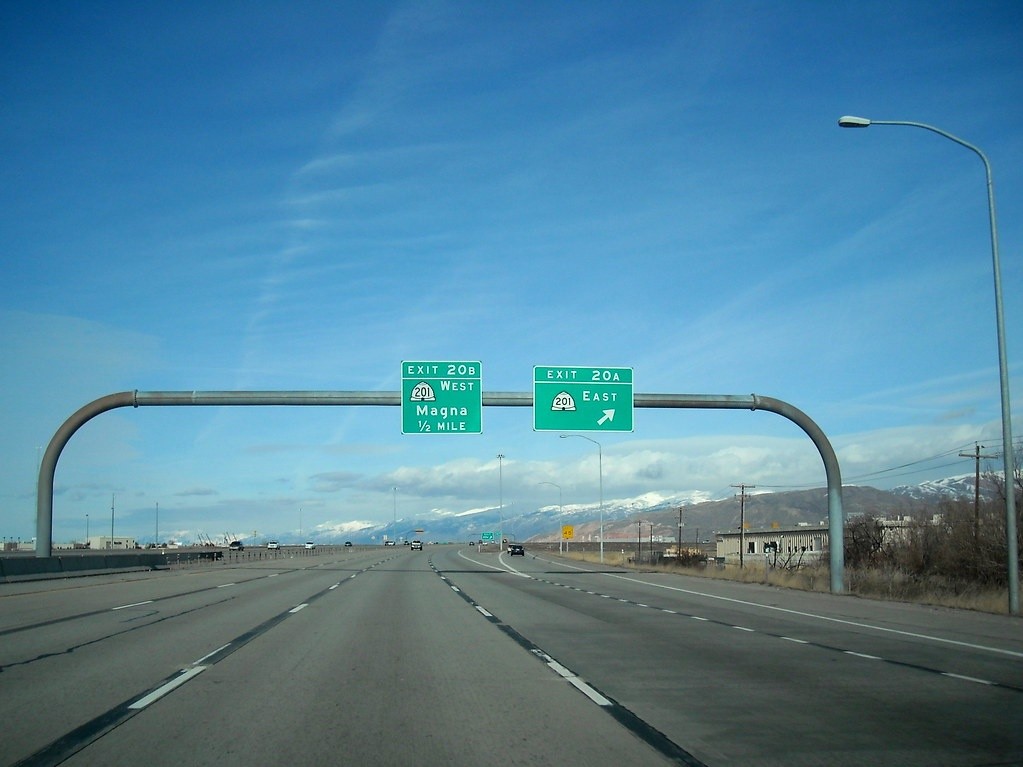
[400,360,483,435]
[533,365,634,433]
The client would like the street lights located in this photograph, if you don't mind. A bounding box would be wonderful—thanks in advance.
[393,487,398,540]
[496,453,505,551]
[560,434,604,564]
[86,514,89,544]
[539,481,562,554]
[299,507,303,543]
[838,116,1020,617]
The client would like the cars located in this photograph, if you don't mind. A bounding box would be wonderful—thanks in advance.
[510,544,525,556]
[491,541,495,544]
[427,541,438,545]
[267,540,280,550]
[479,540,483,544]
[404,540,409,545]
[482,542,488,546]
[229,541,245,551]
[384,540,395,547]
[304,541,315,549]
[503,539,507,543]
[345,542,352,546]
[469,542,474,545]
[507,544,516,553]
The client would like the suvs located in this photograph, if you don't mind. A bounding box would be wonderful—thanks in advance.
[411,540,423,550]
